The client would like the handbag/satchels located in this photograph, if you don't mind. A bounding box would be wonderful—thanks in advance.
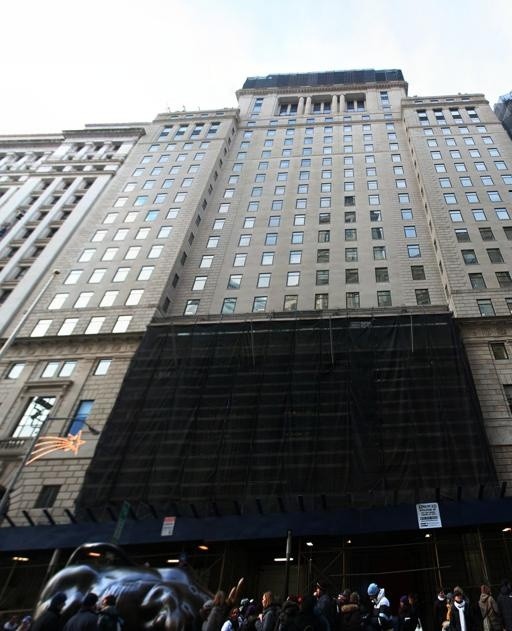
[482,614,492,631]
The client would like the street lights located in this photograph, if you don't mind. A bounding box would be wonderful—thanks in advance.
[1,415,102,521]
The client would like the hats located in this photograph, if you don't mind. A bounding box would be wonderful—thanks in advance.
[367,581,379,595]
[315,575,329,590]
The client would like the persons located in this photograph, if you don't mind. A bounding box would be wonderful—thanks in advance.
[97,595,118,631]
[16,615,31,631]
[64,591,99,631]
[32,591,66,631]
[3,614,20,631]
[221,577,512,631]
[198,591,227,631]
